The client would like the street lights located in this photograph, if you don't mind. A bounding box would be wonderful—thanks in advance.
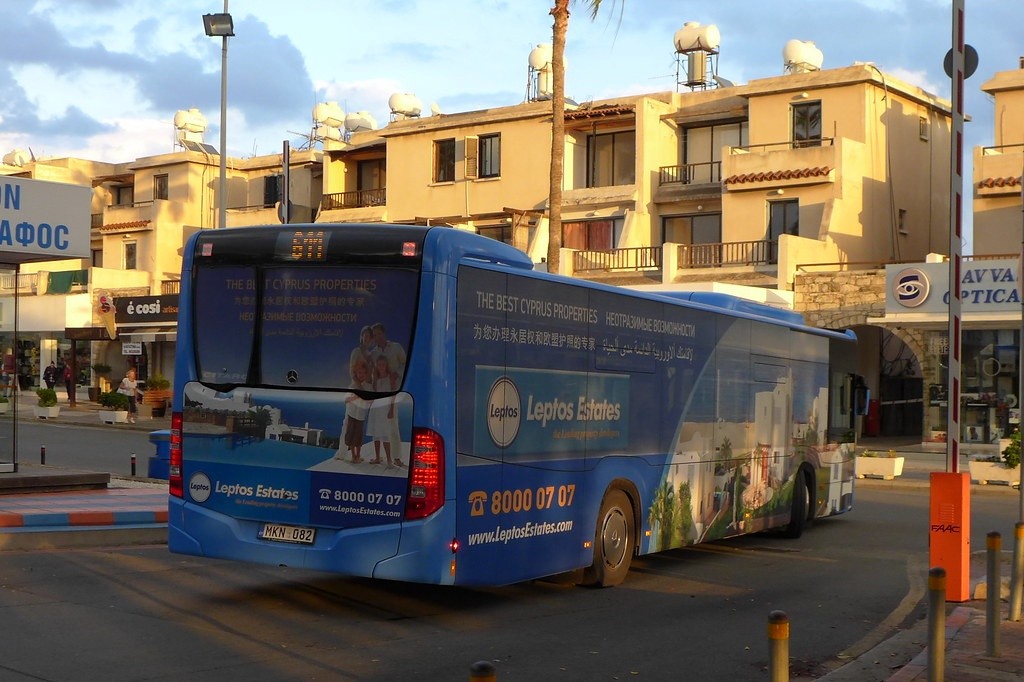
[201,1,236,229]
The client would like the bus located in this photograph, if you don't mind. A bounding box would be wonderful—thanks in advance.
[168,222,871,591]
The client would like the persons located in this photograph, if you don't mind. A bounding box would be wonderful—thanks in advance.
[120,370,137,424]
[43,361,59,390]
[64,363,72,401]
[334,322,409,469]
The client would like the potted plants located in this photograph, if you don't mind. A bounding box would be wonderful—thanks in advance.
[0,394,9,413]
[854,448,904,476]
[137,378,173,410]
[33,387,60,418]
[97,391,130,422]
[967,431,1021,482]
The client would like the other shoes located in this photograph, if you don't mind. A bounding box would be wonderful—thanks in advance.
[334,453,345,460]
[130,417,136,424]
[369,458,381,464]
[352,458,361,463]
[395,458,403,466]
[125,419,128,423]
[387,461,394,469]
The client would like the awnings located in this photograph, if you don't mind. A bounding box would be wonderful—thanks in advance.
[119,327,177,342]
